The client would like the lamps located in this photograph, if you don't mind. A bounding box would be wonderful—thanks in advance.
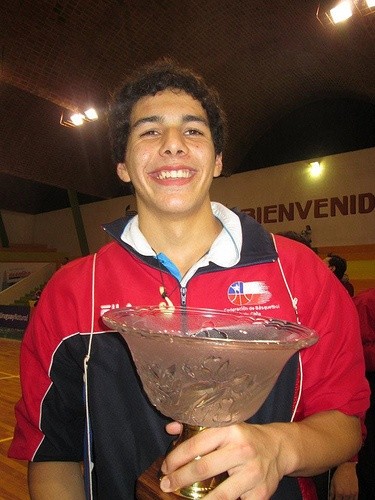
[304,161,324,181]
[59,106,98,127]
[316,0,375,32]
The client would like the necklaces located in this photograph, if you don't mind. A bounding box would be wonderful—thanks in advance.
[150,247,210,318]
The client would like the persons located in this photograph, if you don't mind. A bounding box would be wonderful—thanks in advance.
[7,58,370,500]
[278,230,373,500]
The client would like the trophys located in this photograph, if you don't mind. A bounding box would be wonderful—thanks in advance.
[100,305,319,500]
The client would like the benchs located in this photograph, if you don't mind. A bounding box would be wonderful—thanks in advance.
[9,279,48,306]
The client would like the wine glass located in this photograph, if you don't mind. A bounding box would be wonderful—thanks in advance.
[101,307,317,500]
